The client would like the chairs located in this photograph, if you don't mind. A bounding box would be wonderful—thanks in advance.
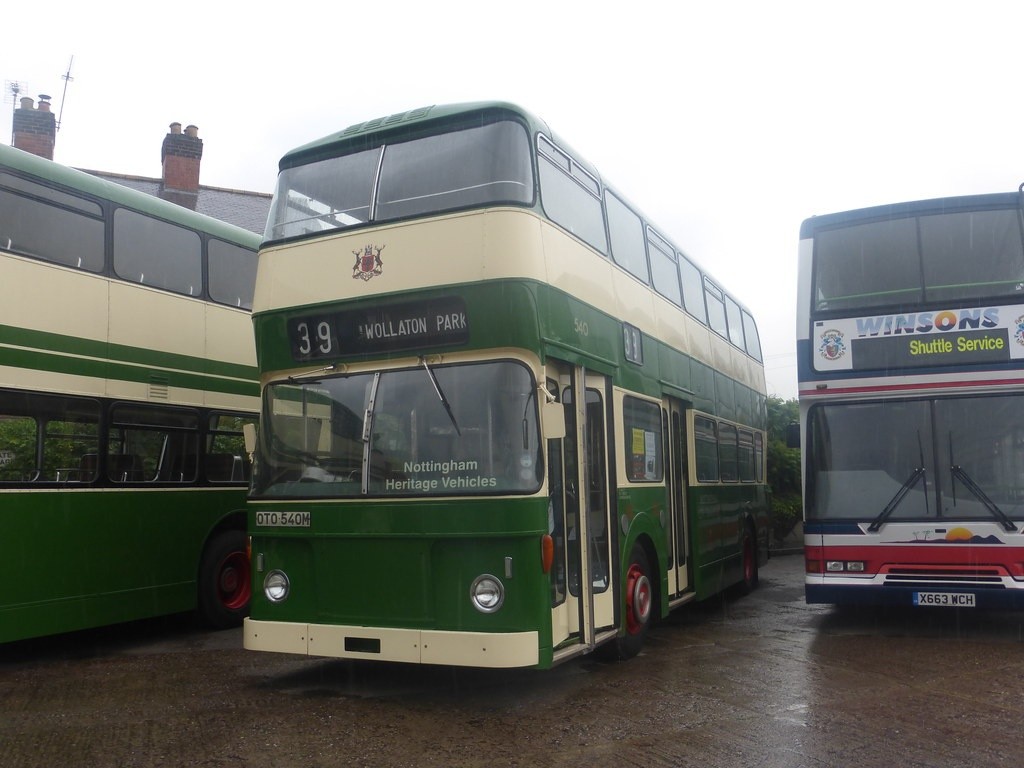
[171,284,195,295]
[0,235,12,248]
[226,296,242,306]
[62,253,82,268]
[128,271,145,284]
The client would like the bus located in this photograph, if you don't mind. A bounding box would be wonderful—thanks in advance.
[242,97,772,673]
[796,181,1024,614]
[0,143,350,646]
